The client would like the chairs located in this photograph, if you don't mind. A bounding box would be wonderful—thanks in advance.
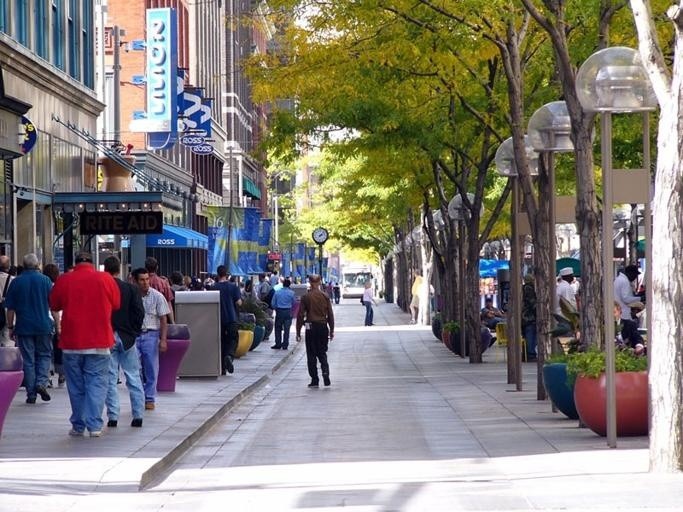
[496,323,527,366]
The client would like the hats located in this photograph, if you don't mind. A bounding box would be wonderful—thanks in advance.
[560,267,573,276]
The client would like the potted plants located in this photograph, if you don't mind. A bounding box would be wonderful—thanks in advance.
[234,296,274,358]
[541,342,648,436]
[432,313,492,356]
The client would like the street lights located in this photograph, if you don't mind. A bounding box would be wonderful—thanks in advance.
[528,98,596,408]
[494,132,546,391]
[575,43,661,450]
[384,191,484,358]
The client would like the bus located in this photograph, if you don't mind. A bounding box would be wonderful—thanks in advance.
[342,267,373,299]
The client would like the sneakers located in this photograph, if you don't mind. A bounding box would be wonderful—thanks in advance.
[26,399,35,403]
[146,401,154,409]
[108,420,117,427]
[69,429,84,436]
[90,431,101,436]
[323,373,330,386]
[308,383,319,387]
[131,419,142,427]
[36,385,50,401]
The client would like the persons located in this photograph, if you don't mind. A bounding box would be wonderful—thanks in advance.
[362,281,378,327]
[296,275,334,386]
[2,256,309,438]
[325,281,340,304]
[409,262,646,353]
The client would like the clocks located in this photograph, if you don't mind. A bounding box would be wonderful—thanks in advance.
[312,227,329,245]
